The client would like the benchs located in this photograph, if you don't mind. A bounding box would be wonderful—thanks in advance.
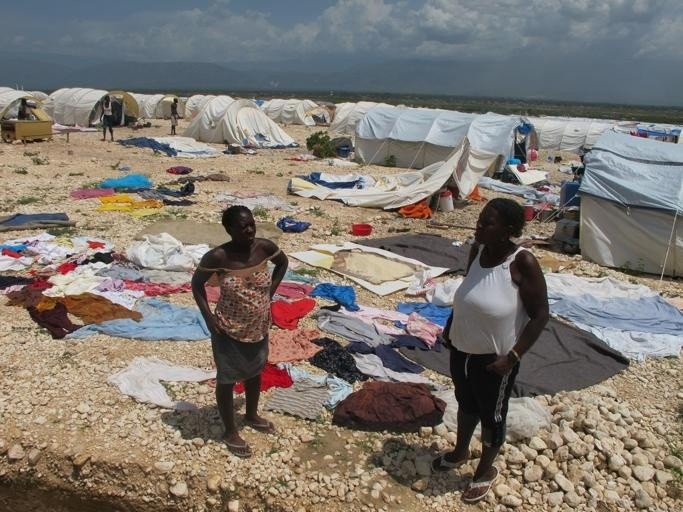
[20,130,69,145]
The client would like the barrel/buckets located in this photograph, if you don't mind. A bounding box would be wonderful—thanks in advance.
[523,205,532,220]
[440,192,454,212]
[336,146,350,159]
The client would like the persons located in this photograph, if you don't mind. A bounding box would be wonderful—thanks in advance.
[430,198,550,505]
[191,206,287,457]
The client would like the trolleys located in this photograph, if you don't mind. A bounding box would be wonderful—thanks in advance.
[0,94,53,144]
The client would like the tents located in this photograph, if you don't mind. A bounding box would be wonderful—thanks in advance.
[285,82,683,286]
[1,84,295,154]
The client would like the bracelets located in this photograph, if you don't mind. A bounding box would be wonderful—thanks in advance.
[509,349,521,364]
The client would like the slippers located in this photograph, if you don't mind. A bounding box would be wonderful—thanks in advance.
[242,418,275,434]
[221,438,252,457]
[430,448,472,472]
[462,464,498,502]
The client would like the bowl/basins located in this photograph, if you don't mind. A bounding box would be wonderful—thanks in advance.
[351,224,372,236]
